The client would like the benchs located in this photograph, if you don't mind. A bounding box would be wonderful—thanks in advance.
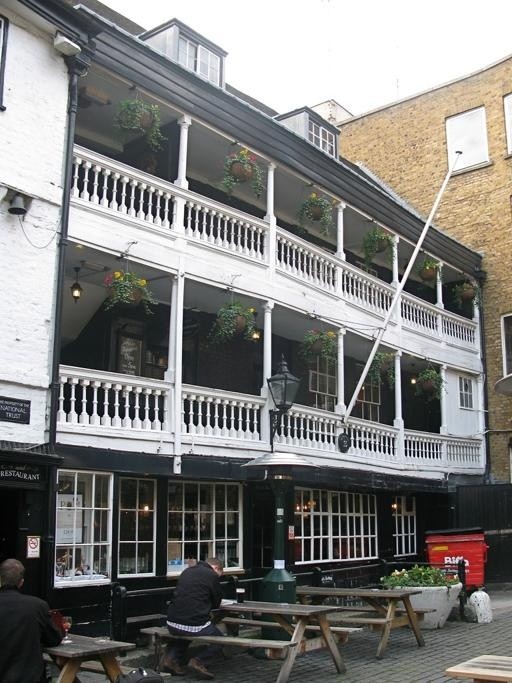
[79,660,171,683]
[139,606,439,682]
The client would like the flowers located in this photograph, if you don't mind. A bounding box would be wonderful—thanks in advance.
[379,563,460,589]
[220,145,339,237]
[296,329,337,363]
[102,271,158,318]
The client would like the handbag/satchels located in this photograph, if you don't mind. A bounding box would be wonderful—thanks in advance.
[116,667,164,683]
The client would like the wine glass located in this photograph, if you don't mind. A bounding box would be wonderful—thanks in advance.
[62,616,74,644]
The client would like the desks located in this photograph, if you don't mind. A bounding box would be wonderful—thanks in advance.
[41,633,135,683]
[217,586,425,682]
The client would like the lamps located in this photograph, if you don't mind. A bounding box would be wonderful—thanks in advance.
[265,351,303,452]
[68,266,82,303]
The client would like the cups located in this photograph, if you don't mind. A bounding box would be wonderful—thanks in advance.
[236,587,247,604]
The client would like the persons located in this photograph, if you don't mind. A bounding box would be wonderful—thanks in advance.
[0,558,63,683]
[162,558,224,678]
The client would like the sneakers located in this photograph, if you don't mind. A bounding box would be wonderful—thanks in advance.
[162,655,186,674]
[188,658,214,678]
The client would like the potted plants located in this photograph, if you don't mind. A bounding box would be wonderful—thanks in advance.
[114,89,167,152]
[364,224,482,307]
[369,351,441,399]
[208,302,258,346]
